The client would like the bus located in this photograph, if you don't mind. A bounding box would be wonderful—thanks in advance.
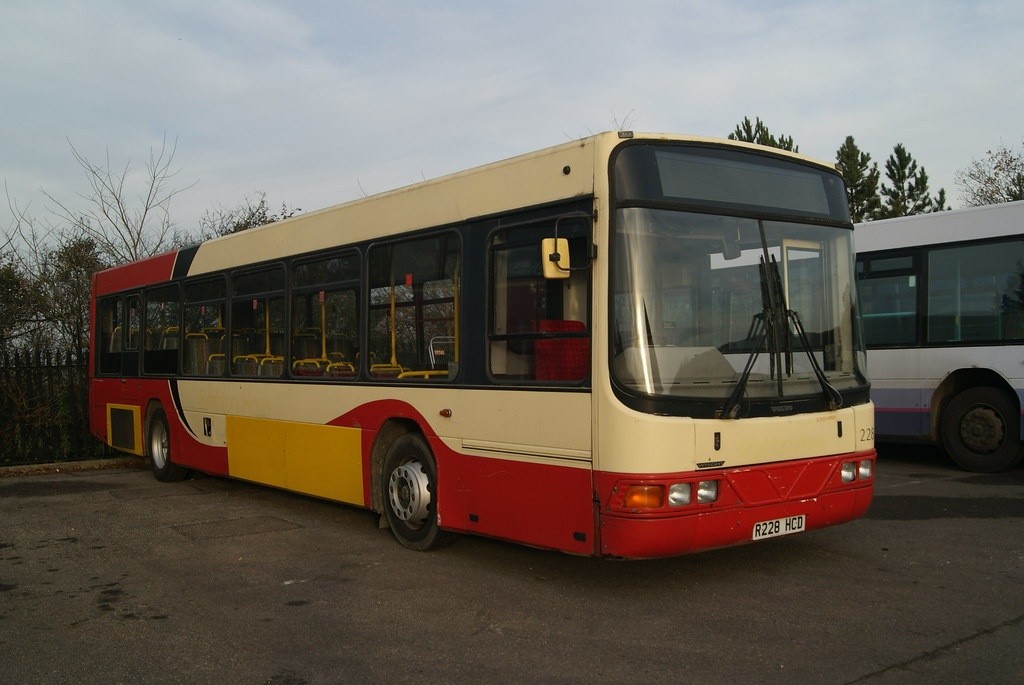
[89,131,877,564]
[708,198,1024,476]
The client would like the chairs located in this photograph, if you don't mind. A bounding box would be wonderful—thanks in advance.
[108,325,457,381]
[531,318,590,382]
